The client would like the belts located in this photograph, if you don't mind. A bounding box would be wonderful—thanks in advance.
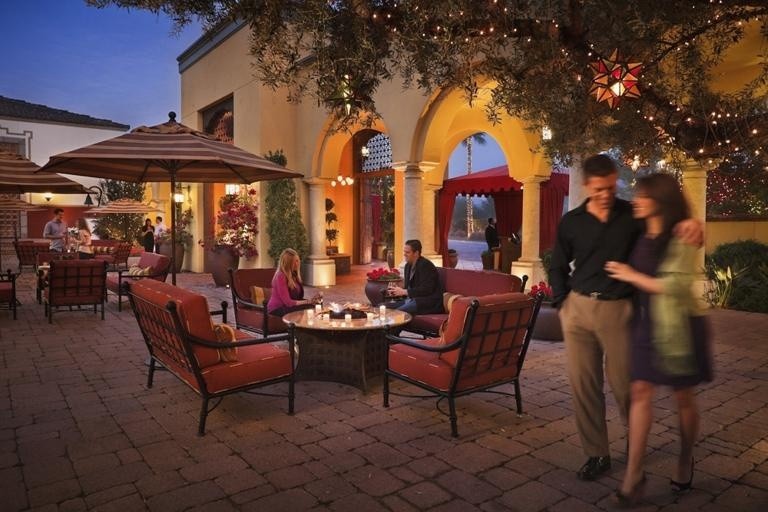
[580,290,611,301]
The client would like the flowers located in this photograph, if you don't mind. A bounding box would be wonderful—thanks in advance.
[527,281,554,302]
[153,206,193,245]
[197,184,259,262]
[366,267,400,281]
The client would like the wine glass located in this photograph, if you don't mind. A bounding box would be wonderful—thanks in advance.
[387,282,398,304]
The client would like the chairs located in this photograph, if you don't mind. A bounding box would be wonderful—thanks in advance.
[0,240,173,323]
[379,290,546,438]
[226,267,315,338]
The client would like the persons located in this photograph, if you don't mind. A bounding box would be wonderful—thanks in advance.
[140,218,155,252]
[550,151,704,481]
[387,239,445,337]
[267,248,324,317]
[602,172,714,508]
[43,208,68,254]
[73,217,92,259]
[153,216,165,254]
[485,217,500,252]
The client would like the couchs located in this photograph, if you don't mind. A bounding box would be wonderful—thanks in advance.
[122,274,296,436]
[380,267,529,337]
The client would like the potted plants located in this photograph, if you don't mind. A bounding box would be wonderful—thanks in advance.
[380,209,394,270]
[325,198,339,255]
[482,251,494,269]
[262,148,312,280]
[449,248,457,267]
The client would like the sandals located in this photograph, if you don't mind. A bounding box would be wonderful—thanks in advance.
[607,471,648,508]
[669,457,696,495]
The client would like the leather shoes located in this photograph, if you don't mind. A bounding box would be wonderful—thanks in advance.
[578,456,610,481]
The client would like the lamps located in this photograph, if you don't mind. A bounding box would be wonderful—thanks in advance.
[84,185,102,208]
[224,183,240,199]
[44,192,52,200]
[174,182,192,208]
[588,48,644,111]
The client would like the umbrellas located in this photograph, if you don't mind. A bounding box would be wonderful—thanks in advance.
[0,147,98,197]
[83,196,167,268]
[32,110,306,287]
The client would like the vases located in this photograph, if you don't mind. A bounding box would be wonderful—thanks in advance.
[527,292,563,341]
[211,245,240,286]
[376,242,388,260]
[159,240,183,273]
[365,277,404,307]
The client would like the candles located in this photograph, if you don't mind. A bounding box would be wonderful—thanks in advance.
[379,305,386,314]
[316,305,321,313]
[367,313,373,321]
[345,314,352,323]
[307,309,313,318]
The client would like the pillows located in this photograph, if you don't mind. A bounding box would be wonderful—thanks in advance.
[249,285,271,310]
[442,292,454,313]
[447,295,464,312]
[214,323,238,362]
